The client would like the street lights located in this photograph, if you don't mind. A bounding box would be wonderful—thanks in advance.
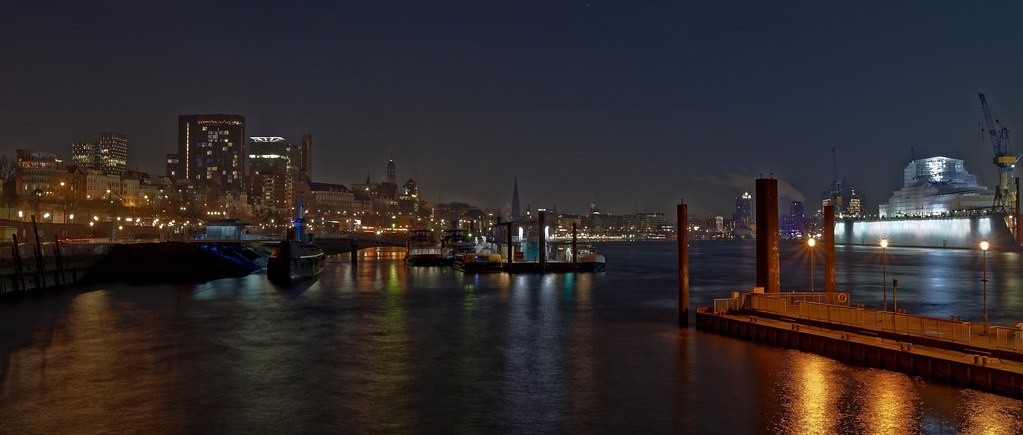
[14,183,826,241]
[808,237,815,293]
[879,238,888,310]
[979,239,991,334]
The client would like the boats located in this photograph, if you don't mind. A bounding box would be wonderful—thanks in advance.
[399,210,605,269]
[832,90,1022,251]
[269,228,326,286]
